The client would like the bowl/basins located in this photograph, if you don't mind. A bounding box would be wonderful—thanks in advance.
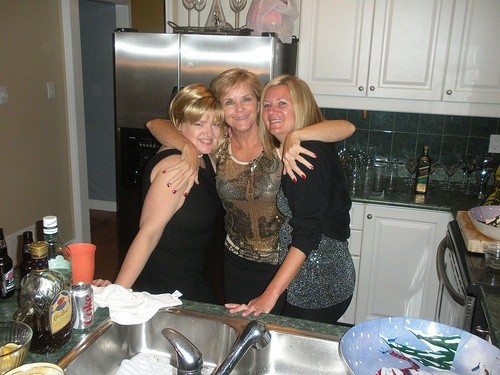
[467,205,500,241]
[3,362,64,375]
[483,241,500,270]
[0,319,33,375]
[477,260,500,286]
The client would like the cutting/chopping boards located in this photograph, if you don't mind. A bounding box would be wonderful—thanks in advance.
[456,210,500,254]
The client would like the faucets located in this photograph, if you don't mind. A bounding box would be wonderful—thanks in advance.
[210,319,272,375]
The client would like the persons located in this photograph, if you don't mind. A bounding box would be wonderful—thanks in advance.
[145,68,356,316]
[221,74,357,325]
[88,82,225,304]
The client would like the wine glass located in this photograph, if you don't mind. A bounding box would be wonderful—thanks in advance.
[406,152,495,203]
[181,0,198,27]
[192,0,207,27]
[229,0,247,32]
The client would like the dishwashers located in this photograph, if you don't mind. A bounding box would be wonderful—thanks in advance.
[434,229,476,332]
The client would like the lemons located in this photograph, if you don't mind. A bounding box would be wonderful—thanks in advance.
[0,343,24,375]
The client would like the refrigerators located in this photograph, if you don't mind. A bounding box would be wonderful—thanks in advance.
[112,28,299,265]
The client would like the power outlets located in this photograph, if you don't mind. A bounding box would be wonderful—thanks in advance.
[488,135,500,153]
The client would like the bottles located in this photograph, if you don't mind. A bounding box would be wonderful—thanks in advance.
[19,241,73,354]
[414,144,432,194]
[338,149,399,198]
[42,215,72,284]
[0,228,15,300]
[20,231,35,278]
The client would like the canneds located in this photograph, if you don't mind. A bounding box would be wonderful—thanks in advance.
[71,282,94,329]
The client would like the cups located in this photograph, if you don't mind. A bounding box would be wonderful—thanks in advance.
[0,258,6,301]
[61,242,97,285]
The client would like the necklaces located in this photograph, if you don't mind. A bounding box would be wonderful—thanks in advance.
[197,153,203,157]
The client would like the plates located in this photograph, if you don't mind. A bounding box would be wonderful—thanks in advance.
[338,316,500,375]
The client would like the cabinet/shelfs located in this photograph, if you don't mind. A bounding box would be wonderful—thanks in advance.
[296,0,455,114]
[442,0,500,119]
[338,202,454,327]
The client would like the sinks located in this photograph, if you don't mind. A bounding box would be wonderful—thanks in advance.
[230,325,354,375]
[55,305,240,375]
